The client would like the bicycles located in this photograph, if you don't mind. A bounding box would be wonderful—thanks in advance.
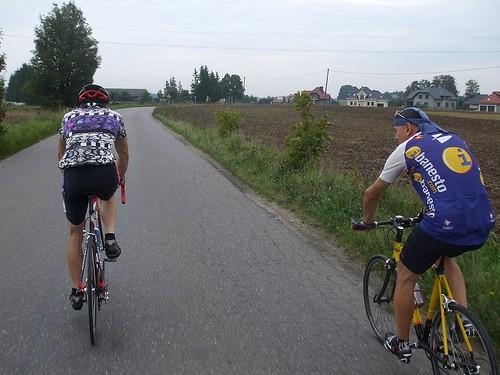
[79,177,126,345]
[353,211,500,375]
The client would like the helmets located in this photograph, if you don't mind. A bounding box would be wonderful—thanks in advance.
[78,84,110,105]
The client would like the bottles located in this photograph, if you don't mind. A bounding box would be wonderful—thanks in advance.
[413,282,424,308]
[94,227,101,251]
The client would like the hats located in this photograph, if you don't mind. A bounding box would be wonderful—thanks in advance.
[393,108,449,134]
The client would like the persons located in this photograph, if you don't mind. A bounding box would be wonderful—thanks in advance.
[358,106,495,362]
[57,83,129,311]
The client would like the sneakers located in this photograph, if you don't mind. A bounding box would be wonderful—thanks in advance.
[450,319,478,343]
[383,333,413,363]
[69,294,84,310]
[104,239,122,260]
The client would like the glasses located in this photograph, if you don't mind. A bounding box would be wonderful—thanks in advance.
[394,109,405,120]
